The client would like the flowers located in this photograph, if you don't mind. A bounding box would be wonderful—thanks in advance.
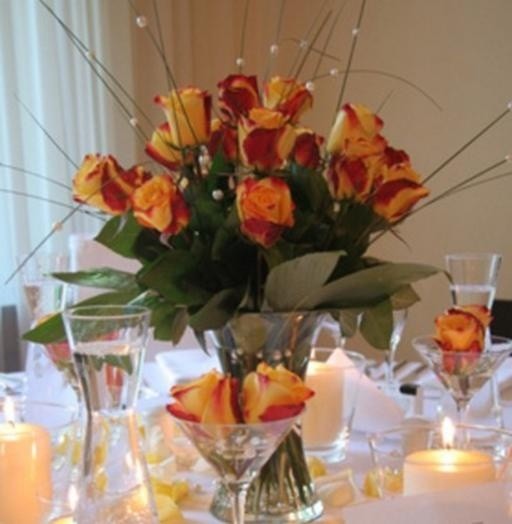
[0,1,512,510]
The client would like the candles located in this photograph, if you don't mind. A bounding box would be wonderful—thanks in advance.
[400,445,496,497]
[300,359,343,450]
[3,423,53,522]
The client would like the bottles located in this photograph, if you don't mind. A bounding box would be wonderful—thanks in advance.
[61,304,152,524]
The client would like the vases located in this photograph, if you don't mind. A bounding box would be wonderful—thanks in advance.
[205,312,328,524]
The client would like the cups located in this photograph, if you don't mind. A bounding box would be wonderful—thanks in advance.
[446,252,502,341]
[368,423,512,495]
[21,252,75,321]
[20,324,82,429]
[1,399,76,524]
[301,347,367,460]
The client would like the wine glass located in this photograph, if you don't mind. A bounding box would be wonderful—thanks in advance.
[170,406,302,524]
[410,335,512,422]
[314,311,406,382]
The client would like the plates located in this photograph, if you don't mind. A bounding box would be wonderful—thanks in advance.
[157,348,223,381]
